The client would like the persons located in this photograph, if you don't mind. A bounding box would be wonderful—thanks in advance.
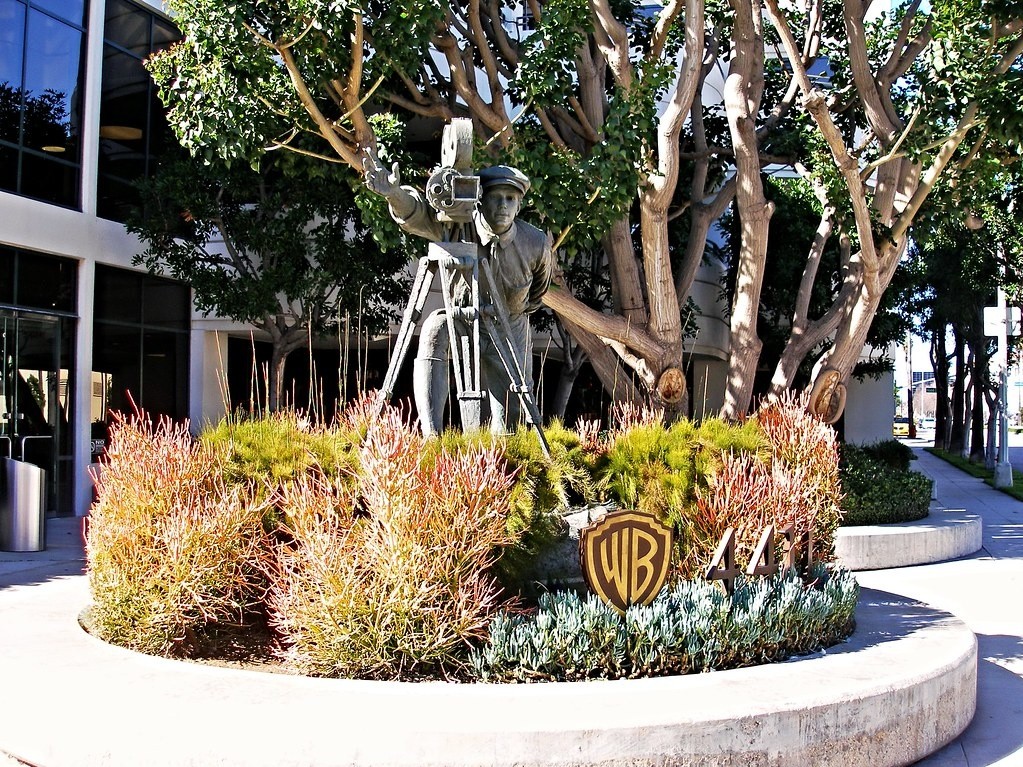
[362,147,552,438]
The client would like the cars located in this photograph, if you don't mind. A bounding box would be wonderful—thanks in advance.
[894,417,909,438]
[919,418,935,429]
